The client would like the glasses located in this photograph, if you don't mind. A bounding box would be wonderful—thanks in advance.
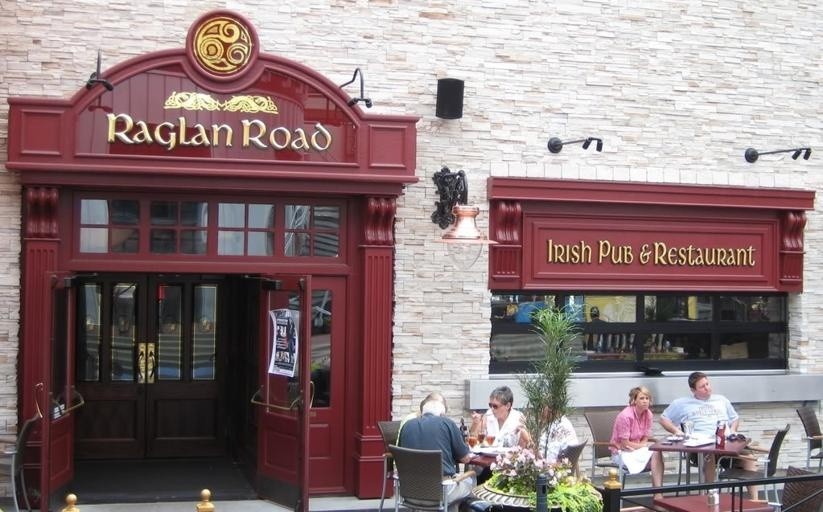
[487,403,504,409]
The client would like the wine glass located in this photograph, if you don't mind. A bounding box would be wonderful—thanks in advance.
[469,432,496,451]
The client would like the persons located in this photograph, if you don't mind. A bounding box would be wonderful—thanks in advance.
[464,386,530,488]
[607,387,667,501]
[658,371,760,500]
[589,306,608,322]
[392,393,448,487]
[513,402,584,486]
[397,400,480,512]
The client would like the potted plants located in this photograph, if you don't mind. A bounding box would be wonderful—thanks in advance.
[470,303,604,512]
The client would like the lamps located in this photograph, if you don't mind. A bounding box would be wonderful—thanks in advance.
[746,147,810,161]
[86,48,114,92]
[548,138,603,152]
[339,68,372,108]
[432,165,497,271]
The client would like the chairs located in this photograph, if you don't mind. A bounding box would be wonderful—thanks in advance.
[377,419,401,511]
[0,413,42,512]
[716,423,793,503]
[795,410,822,473]
[748,465,821,511]
[554,436,590,483]
[676,423,723,495]
[585,409,662,509]
[388,444,478,511]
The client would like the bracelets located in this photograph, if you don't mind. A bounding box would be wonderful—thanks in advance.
[525,442,534,450]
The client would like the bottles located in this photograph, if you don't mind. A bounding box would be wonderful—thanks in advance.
[715,420,727,449]
[460,417,467,444]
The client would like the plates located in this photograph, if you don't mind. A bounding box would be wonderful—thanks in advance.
[482,448,511,457]
[667,433,715,447]
